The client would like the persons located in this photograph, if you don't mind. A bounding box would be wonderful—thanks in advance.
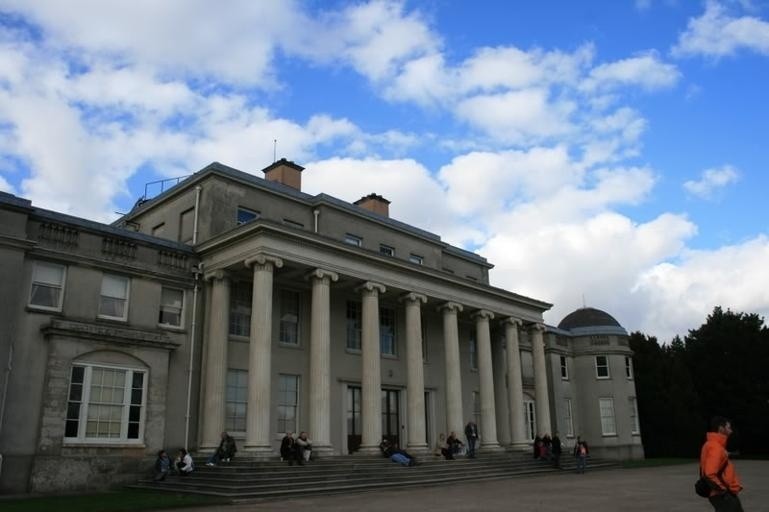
[296,431,317,464]
[532,429,563,470]
[279,429,304,467]
[203,430,238,467]
[465,416,480,459]
[689,412,745,511]
[573,434,589,474]
[154,448,172,481]
[437,432,456,460]
[379,434,415,467]
[447,430,466,456]
[172,446,196,479]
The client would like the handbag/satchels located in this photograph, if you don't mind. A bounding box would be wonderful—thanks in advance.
[694,478,713,498]
